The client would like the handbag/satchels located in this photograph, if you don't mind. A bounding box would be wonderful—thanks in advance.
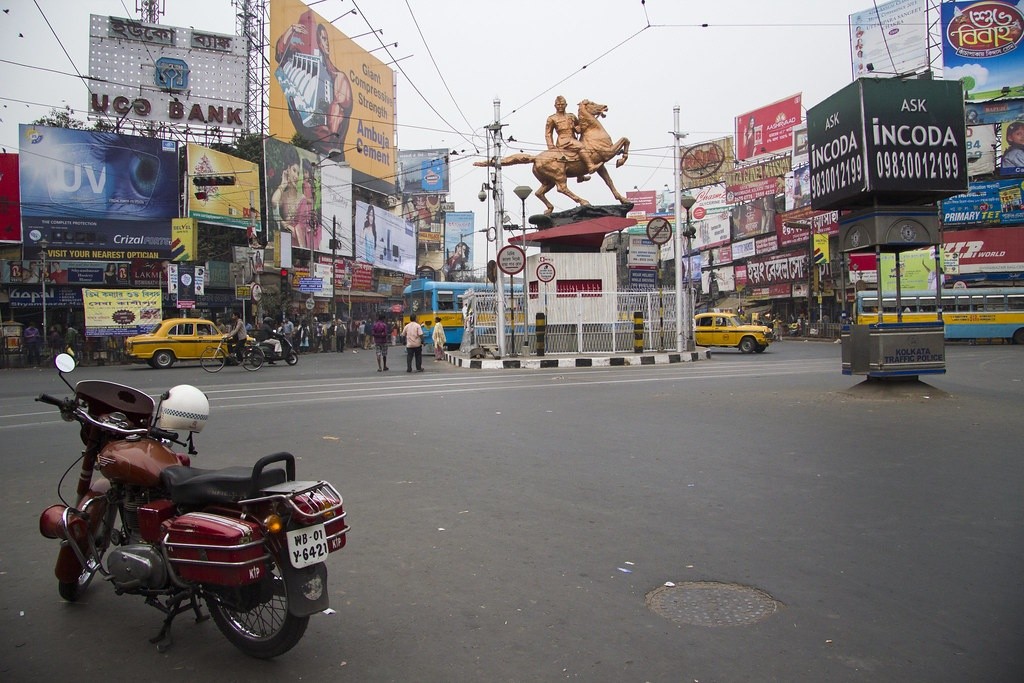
[433,345,444,360]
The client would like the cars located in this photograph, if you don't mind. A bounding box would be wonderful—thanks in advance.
[124,318,257,370]
[694,312,775,354]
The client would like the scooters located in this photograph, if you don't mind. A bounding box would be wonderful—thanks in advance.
[248,328,299,368]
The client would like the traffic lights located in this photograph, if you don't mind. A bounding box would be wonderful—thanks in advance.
[194,192,207,200]
[193,175,236,186]
[279,268,289,294]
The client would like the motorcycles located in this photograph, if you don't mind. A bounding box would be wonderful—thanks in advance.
[33,352,353,661]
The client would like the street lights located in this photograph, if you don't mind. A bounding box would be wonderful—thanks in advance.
[308,149,351,333]
[478,182,508,359]
[680,195,697,340]
[461,227,487,282]
[38,238,50,341]
[512,185,534,346]
[784,221,813,323]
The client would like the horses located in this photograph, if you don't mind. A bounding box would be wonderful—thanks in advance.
[473,99,631,215]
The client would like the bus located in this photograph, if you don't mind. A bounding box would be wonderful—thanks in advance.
[401,276,525,351]
[847,286,1024,345]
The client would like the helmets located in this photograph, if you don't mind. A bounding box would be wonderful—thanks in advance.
[264,317,274,323]
[158,383,209,433]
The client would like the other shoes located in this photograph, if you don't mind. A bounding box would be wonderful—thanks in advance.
[237,361,245,366]
[377,368,381,372]
[268,360,275,364]
[417,367,425,372]
[228,354,236,358]
[383,366,389,371]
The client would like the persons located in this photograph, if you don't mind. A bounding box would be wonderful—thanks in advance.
[966,110,978,125]
[432,317,446,362]
[102,263,118,285]
[1002,121,1024,168]
[363,205,378,264]
[50,263,68,283]
[20,318,228,366]
[252,251,262,272]
[446,241,470,270]
[260,317,282,364]
[709,308,837,344]
[215,317,404,354]
[372,316,389,372]
[269,147,320,249]
[401,313,426,372]
[545,95,603,183]
[223,312,248,365]
[22,262,39,283]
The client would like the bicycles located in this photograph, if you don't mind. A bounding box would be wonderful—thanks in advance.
[200,334,266,373]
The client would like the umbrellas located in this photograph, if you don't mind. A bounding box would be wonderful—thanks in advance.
[385,304,404,319]
